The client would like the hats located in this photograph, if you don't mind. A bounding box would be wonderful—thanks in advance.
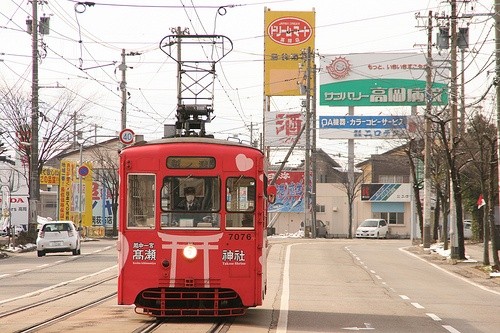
[185,187,195,193]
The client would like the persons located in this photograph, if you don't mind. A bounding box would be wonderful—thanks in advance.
[172,186,202,227]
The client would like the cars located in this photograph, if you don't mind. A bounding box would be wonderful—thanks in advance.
[356,218,390,239]
[36,221,83,257]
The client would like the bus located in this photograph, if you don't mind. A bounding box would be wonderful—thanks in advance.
[116,34,277,322]
